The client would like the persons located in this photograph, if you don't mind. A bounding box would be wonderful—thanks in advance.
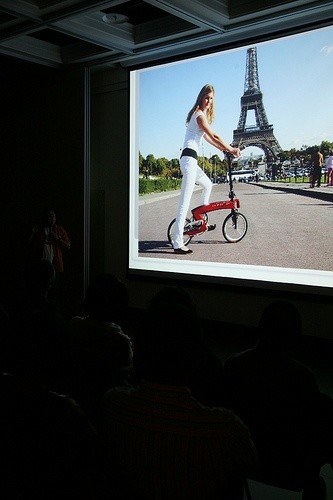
[29,207,72,306]
[145,287,225,408]
[171,84,240,254]
[0,328,98,500]
[308,146,323,188]
[56,271,136,425]
[98,328,259,499]
[325,150,333,187]
[221,298,333,500]
[11,257,68,340]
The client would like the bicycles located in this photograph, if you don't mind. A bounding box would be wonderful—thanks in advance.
[167,146,249,249]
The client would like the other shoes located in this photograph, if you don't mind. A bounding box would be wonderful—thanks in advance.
[174,246,193,254]
[207,224,216,231]
